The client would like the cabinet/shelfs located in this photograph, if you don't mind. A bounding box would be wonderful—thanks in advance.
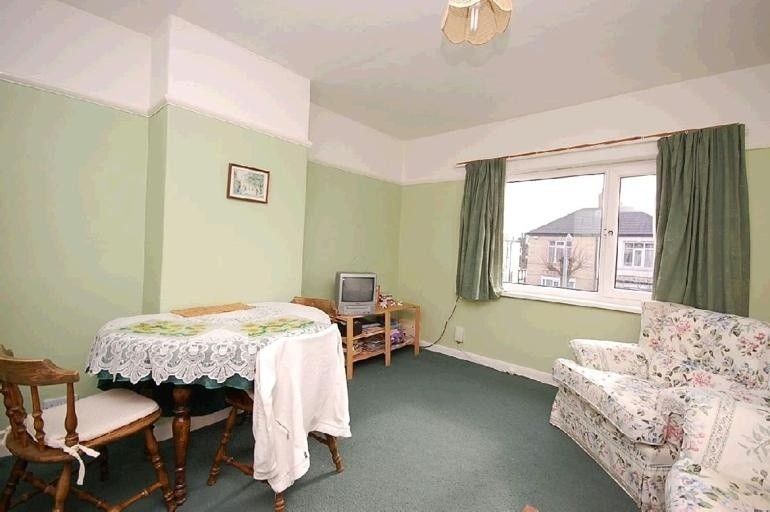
[334,301,421,380]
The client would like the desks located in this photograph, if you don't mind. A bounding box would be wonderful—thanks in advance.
[85,301,332,505]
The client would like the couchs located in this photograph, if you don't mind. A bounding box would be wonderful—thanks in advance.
[665,388,770,512]
[548,298,770,512]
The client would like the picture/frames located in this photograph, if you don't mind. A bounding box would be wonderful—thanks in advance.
[227,163,271,205]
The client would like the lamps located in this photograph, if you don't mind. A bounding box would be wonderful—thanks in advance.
[441,1,513,47]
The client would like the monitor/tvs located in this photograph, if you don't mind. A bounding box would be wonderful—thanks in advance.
[339,273,376,316]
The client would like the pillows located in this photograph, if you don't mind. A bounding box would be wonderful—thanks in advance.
[678,390,770,492]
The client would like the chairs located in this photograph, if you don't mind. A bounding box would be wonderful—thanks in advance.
[207,325,345,512]
[290,294,338,324]
[0,344,178,512]
